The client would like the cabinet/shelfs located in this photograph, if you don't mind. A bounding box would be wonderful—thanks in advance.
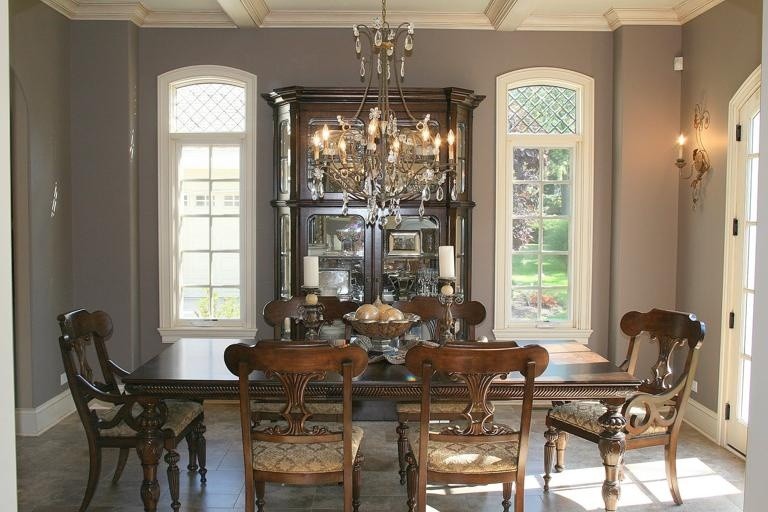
[259,85,487,342]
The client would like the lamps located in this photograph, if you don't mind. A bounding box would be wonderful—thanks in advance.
[310,0,458,226]
[676,104,706,212]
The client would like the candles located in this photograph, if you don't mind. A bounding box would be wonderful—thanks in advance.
[303,255,319,286]
[438,245,455,278]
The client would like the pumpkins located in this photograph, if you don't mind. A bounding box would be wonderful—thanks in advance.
[355,303,404,322]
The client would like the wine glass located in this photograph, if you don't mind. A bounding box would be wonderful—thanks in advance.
[342,310,422,353]
